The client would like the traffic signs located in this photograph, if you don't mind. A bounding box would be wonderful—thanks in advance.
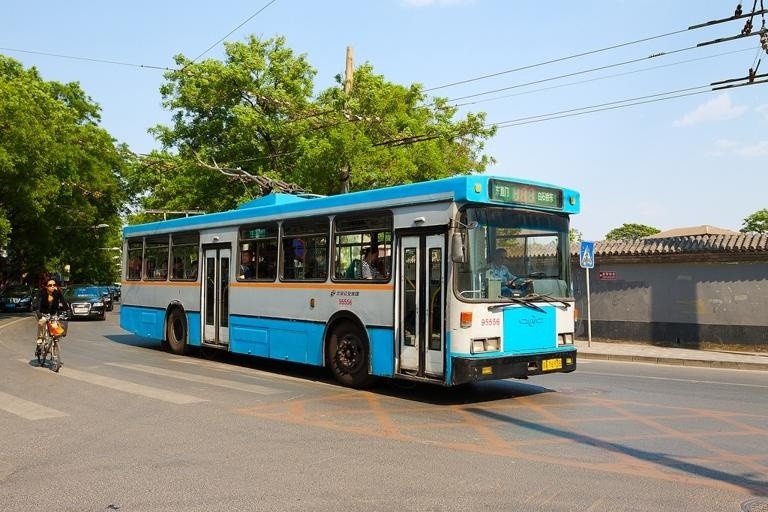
[579,242,594,268]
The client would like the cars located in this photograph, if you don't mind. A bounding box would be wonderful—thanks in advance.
[0,284,34,313]
[62,282,120,322]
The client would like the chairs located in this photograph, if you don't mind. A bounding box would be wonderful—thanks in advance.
[353,261,361,278]
[185,269,192,278]
[475,267,487,280]
[154,266,160,278]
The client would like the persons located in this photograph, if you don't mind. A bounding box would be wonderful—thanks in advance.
[34,276,73,357]
[485,247,532,298]
[128,221,391,282]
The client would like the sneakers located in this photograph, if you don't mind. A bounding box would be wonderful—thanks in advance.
[36,338,42,344]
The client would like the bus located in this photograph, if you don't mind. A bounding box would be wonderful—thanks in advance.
[119,174,581,389]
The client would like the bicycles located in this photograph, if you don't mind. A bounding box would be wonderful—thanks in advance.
[36,311,68,373]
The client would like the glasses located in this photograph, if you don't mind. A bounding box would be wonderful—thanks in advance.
[46,284,56,288]
